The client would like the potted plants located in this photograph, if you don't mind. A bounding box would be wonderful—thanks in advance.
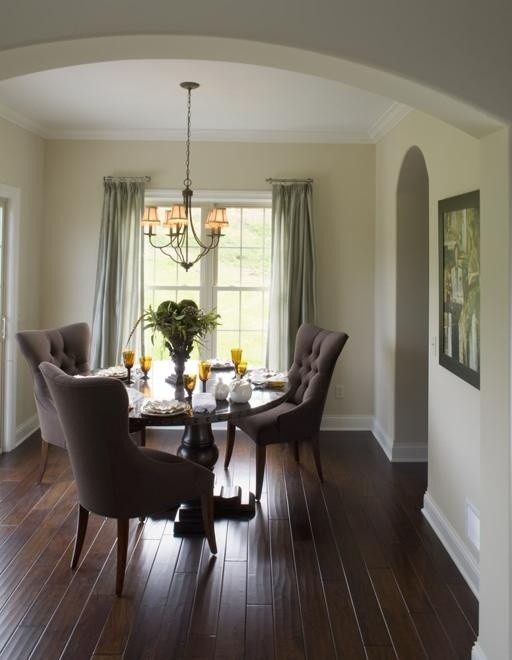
[122,300,221,385]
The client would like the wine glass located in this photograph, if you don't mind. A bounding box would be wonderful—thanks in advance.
[183,374,197,401]
[198,362,211,393]
[139,357,152,379]
[231,348,247,379]
[123,351,136,383]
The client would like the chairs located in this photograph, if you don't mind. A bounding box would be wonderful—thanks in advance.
[38,362,217,595]
[13,322,146,484]
[224,321,349,500]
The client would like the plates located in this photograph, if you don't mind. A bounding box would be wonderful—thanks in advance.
[140,399,191,417]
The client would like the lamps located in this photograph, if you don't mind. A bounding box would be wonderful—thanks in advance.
[137,80,230,273]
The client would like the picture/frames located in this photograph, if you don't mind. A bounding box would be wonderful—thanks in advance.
[437,189,480,389]
